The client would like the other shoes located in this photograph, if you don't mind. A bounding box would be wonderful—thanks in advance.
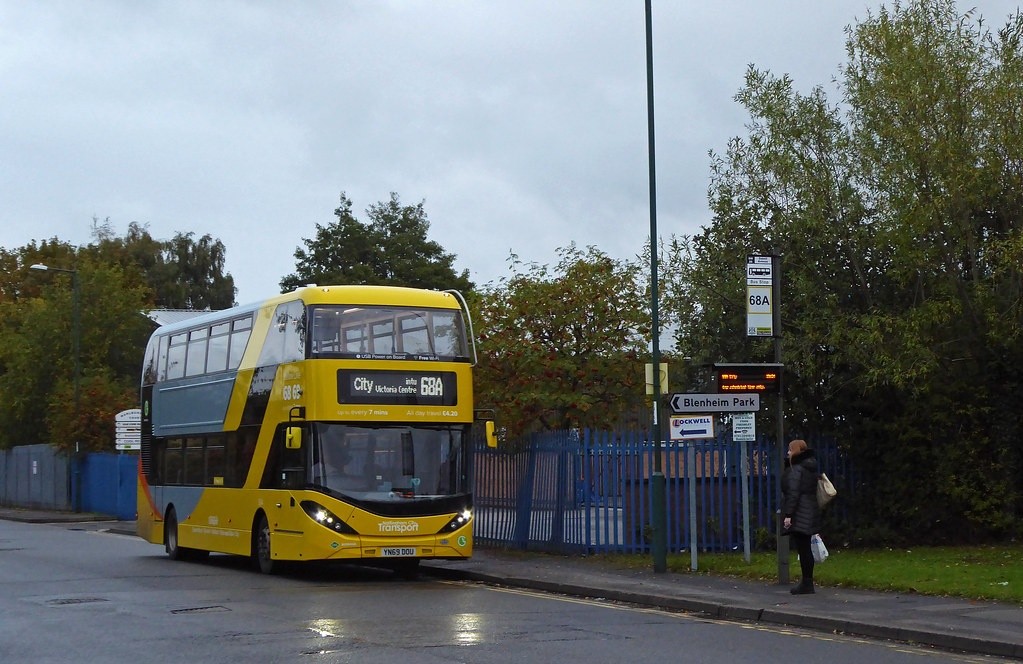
[790,577,816,595]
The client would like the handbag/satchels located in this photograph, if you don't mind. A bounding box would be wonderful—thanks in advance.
[797,534,829,564]
[816,472,837,510]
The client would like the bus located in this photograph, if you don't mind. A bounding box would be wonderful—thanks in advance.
[135,283,499,579]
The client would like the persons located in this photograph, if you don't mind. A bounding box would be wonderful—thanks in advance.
[779,440,821,595]
[311,442,344,477]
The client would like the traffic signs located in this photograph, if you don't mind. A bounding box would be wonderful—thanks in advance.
[669,391,761,414]
[667,414,717,442]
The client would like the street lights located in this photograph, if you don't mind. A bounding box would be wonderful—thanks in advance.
[29,261,83,515]
